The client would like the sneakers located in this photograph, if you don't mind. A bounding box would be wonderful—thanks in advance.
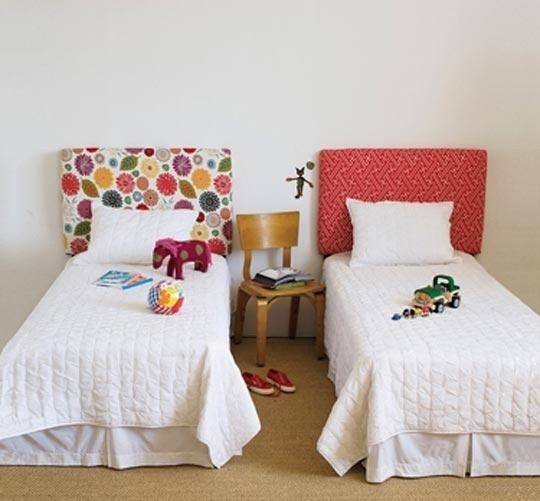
[242,371,274,396]
[266,368,297,393]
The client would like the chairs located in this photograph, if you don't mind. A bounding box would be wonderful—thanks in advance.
[233,210,328,370]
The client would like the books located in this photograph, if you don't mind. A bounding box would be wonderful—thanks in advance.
[94,274,155,291]
[91,270,141,288]
[246,266,316,289]
[270,281,308,291]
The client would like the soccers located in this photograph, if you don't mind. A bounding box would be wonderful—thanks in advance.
[149,279,185,314]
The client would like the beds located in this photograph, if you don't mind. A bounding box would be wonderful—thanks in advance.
[316,148,540,486]
[0,146,265,473]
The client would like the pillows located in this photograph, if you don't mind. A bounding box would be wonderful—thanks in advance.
[85,198,200,268]
[342,194,459,270]
